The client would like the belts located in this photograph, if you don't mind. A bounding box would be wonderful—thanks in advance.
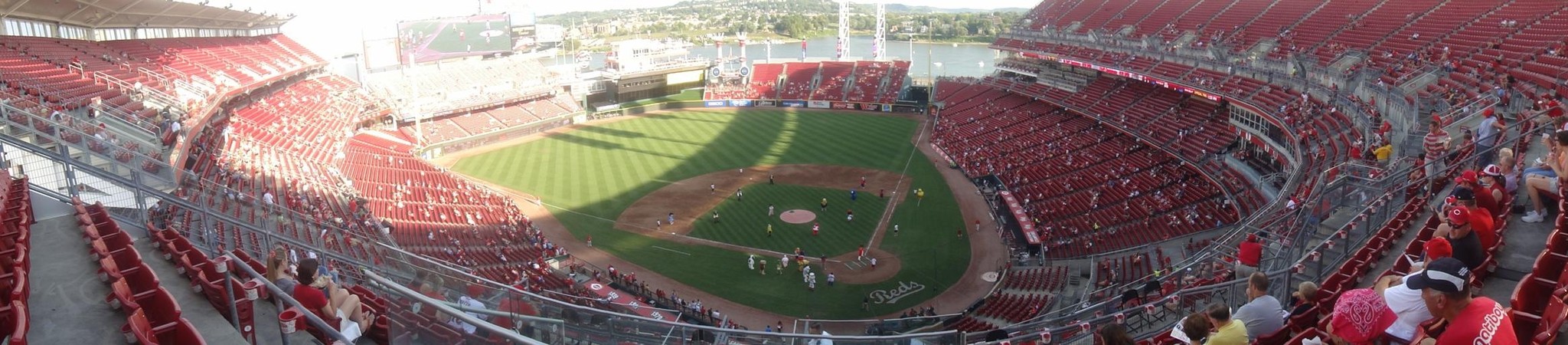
[1242,263,1257,268]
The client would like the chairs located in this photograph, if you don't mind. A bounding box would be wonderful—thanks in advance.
[1247,172,1568,342]
[1,165,496,344]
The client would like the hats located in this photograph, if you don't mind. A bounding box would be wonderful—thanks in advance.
[1445,207,1471,226]
[1449,188,1476,204]
[1541,94,1552,98]
[1479,164,1501,176]
[1455,171,1478,184]
[1406,257,1470,295]
[1422,237,1452,261]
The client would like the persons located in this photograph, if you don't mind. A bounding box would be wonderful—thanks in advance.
[821,198,827,213]
[737,187,743,201]
[860,176,866,190]
[712,209,719,222]
[269,235,941,342]
[850,188,857,201]
[914,189,917,196]
[880,190,884,199]
[768,204,774,216]
[928,11,1568,345]
[711,182,715,193]
[813,222,819,235]
[711,61,909,105]
[739,167,743,175]
[668,213,674,226]
[1,26,570,276]
[656,217,662,231]
[894,222,899,237]
[769,173,774,185]
[767,222,772,235]
[847,209,853,221]
[916,188,924,206]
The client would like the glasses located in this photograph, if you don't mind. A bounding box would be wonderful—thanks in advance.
[1447,222,1468,229]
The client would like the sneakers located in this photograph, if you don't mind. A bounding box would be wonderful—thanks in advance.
[1521,208,1549,223]
